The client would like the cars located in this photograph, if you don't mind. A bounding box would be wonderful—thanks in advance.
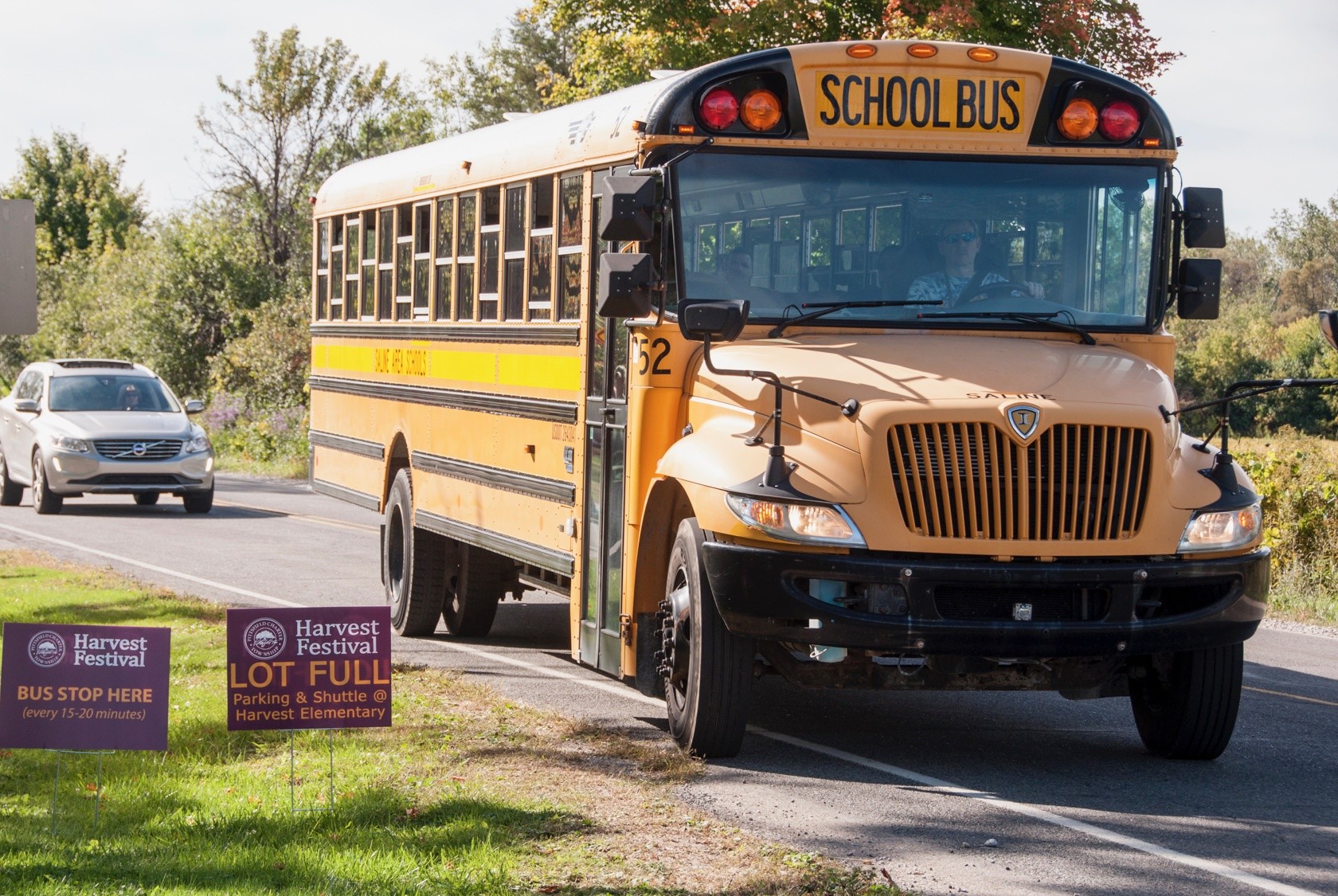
[0,357,215,516]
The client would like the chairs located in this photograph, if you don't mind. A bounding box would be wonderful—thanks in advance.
[49,383,76,411]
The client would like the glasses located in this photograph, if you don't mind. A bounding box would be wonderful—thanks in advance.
[945,232,978,242]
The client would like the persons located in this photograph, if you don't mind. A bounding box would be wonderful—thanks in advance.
[723,250,755,287]
[116,384,141,410]
[907,216,1046,308]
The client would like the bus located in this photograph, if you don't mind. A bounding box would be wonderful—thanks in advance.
[304,39,1273,768]
